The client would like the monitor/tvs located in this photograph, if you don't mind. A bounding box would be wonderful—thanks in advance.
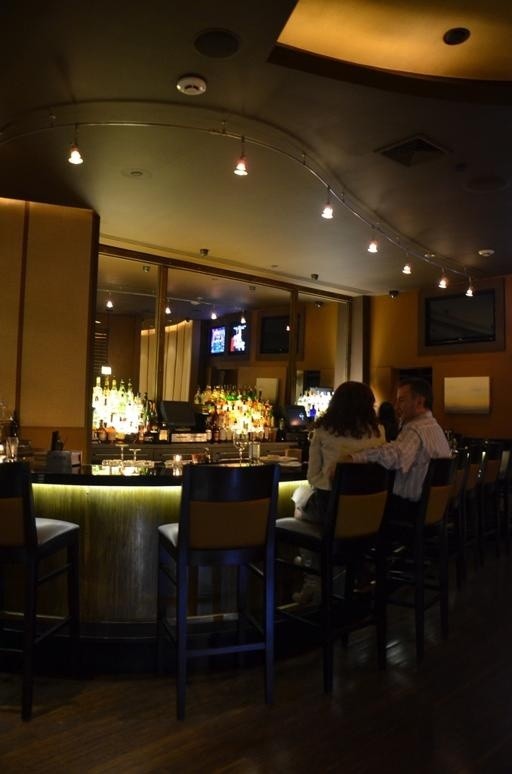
[209,321,251,356]
[417,277,506,357]
[159,401,197,427]
[275,406,308,428]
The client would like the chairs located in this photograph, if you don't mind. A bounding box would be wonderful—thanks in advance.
[344,444,512,665]
[276,461,401,700]
[1,460,81,721]
[156,462,279,719]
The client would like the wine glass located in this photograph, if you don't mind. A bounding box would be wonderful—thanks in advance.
[232,430,249,467]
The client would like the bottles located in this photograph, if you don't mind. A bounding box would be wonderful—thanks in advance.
[97,419,107,442]
[158,422,170,441]
[92,375,151,426]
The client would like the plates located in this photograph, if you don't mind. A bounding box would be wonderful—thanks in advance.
[259,456,303,467]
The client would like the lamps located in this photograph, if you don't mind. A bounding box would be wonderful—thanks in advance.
[56,119,482,299]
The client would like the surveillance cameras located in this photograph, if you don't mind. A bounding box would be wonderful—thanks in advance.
[313,301,323,308]
[389,290,399,299]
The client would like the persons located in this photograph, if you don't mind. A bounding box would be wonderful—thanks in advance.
[337,377,452,594]
[378,401,400,443]
[288,379,386,604]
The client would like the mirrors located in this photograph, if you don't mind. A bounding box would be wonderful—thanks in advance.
[96,252,353,444]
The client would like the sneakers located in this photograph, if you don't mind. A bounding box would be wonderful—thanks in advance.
[291,577,322,604]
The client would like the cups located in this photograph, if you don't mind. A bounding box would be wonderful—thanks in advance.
[4,436,19,460]
[248,441,261,467]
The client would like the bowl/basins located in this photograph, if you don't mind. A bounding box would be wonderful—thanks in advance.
[121,466,135,477]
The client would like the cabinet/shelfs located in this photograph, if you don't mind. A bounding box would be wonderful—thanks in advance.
[0,195,94,429]
